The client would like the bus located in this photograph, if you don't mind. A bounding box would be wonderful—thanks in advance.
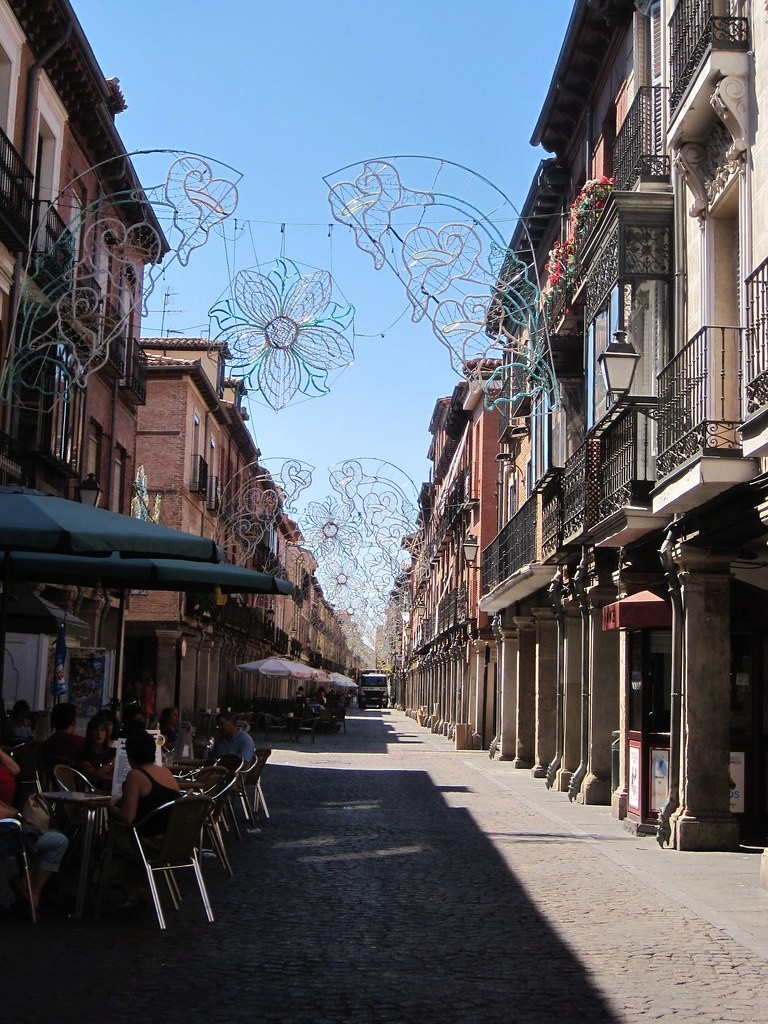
[356,672,389,709]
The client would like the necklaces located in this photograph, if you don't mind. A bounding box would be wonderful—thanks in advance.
[17,720,24,728]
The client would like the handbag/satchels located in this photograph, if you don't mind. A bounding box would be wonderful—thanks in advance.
[22,793,51,833]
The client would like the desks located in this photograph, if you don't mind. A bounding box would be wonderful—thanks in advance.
[281,715,303,741]
[40,788,112,922]
[174,757,207,767]
[178,781,206,870]
[168,765,195,781]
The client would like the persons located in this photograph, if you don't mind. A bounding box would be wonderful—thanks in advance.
[0,676,254,920]
[295,686,346,731]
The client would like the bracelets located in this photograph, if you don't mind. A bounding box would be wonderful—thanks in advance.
[108,762,114,768]
[17,808,23,818]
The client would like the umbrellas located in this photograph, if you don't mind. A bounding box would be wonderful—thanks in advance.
[0,484,225,695]
[0,551,304,710]
[235,654,359,701]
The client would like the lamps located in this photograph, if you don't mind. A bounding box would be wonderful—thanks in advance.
[264,608,275,630]
[405,625,415,642]
[597,332,657,402]
[494,452,511,462]
[510,426,528,438]
[302,638,311,651]
[417,601,431,621]
[463,533,483,572]
[76,473,103,509]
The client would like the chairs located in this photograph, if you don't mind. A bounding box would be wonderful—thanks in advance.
[0,709,347,930]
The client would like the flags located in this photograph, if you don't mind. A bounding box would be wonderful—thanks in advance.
[50,623,67,696]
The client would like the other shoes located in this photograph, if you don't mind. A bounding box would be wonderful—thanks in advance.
[111,886,146,913]
[9,877,41,921]
